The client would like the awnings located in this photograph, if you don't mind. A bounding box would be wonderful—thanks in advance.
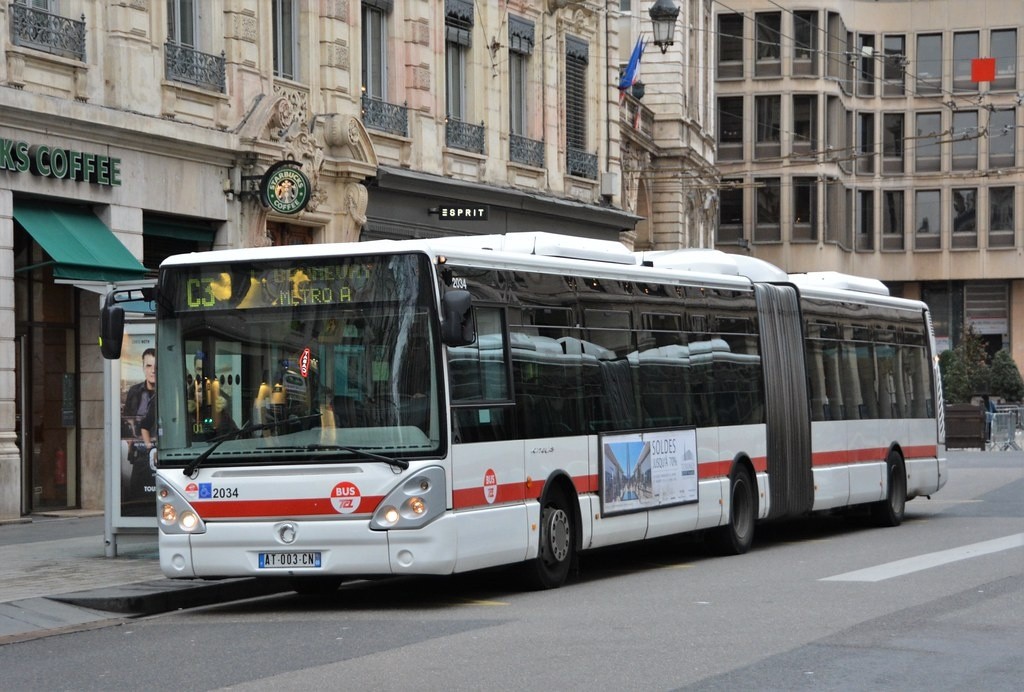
[12,198,152,281]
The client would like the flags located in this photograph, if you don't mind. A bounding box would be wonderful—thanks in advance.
[618,31,649,90]
[970,58,996,82]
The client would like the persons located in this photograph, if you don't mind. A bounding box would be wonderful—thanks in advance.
[185,353,203,408]
[121,349,157,517]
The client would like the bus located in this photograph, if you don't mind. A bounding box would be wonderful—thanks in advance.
[97,230,952,590]
